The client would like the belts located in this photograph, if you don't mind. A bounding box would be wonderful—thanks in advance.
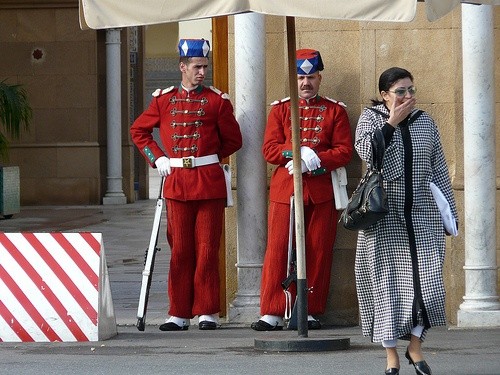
[169,154,220,168]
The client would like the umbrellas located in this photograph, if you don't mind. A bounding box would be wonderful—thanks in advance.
[79,1,500,336]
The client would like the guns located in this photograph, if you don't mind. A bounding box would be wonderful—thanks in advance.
[136,175,166,332]
[282,188,297,329]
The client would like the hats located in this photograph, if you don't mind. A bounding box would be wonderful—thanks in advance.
[178,39,210,58]
[296,49,324,75]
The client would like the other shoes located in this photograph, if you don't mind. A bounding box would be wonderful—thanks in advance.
[405,347,432,375]
[384,368,399,375]
[251,320,284,331]
[199,321,216,330]
[159,322,189,331]
[307,321,321,329]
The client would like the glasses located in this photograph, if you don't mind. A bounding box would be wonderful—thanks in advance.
[385,85,417,98]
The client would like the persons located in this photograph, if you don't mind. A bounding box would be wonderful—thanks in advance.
[130,39,243,331]
[355,67,458,375]
[251,49,353,331]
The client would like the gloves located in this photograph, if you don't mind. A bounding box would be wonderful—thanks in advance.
[285,159,309,175]
[300,145,322,172]
[154,156,172,177]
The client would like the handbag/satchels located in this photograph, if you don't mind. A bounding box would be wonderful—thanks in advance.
[337,129,389,230]
[332,166,350,210]
[219,163,234,207]
[429,181,458,236]
